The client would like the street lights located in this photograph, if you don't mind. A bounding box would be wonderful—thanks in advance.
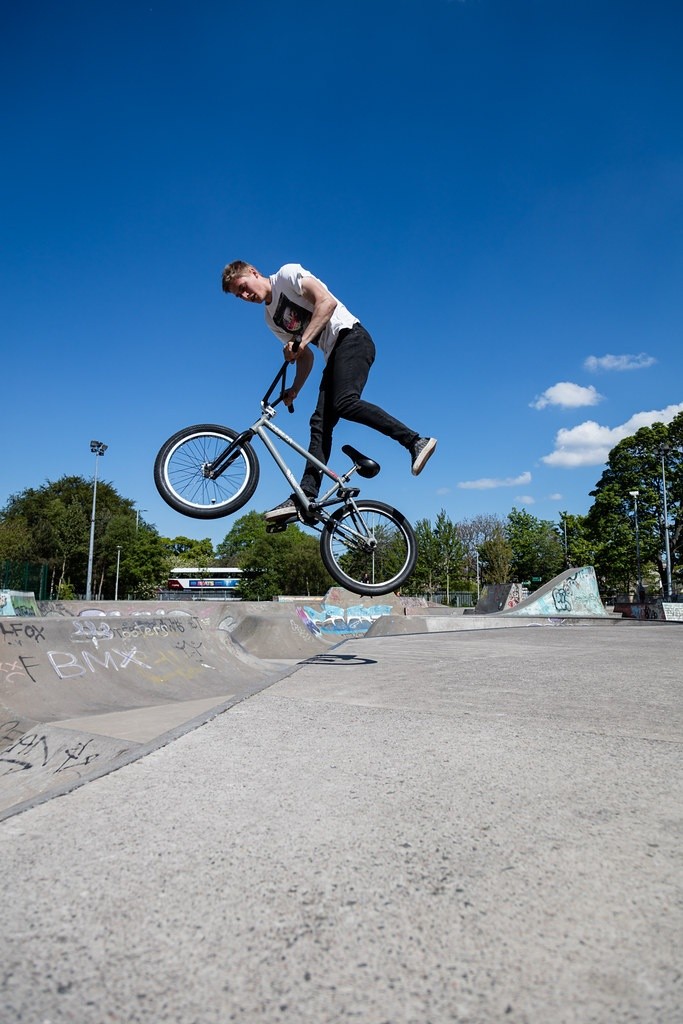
[136,509,148,533]
[630,491,641,585]
[85,441,109,600]
[114,545,123,600]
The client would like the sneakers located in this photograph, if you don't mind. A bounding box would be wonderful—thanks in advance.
[265,497,315,522]
[410,437,438,475]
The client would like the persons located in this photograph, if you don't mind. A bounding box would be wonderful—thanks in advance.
[220,260,438,518]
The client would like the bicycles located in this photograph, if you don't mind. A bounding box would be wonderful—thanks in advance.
[153,332,420,597]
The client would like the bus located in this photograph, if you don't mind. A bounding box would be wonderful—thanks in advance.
[167,568,269,594]
